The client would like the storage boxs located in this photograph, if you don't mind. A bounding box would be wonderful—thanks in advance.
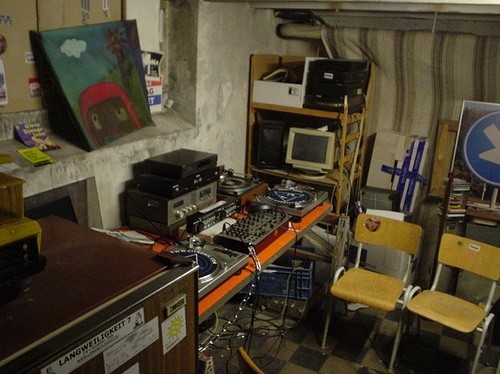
[366,131,430,191]
[253,56,329,109]
[239,246,316,300]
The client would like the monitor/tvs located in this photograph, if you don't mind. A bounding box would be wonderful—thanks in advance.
[286,125,335,174]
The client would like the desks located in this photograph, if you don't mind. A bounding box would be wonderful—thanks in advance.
[112,202,333,324]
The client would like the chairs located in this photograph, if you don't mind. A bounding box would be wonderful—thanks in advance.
[320,213,500,374]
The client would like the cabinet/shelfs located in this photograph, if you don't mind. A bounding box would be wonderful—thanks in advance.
[246,54,374,279]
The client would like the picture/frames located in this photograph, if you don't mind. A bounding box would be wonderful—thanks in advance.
[428,120,500,206]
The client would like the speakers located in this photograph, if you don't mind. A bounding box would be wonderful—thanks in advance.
[256,120,286,170]
